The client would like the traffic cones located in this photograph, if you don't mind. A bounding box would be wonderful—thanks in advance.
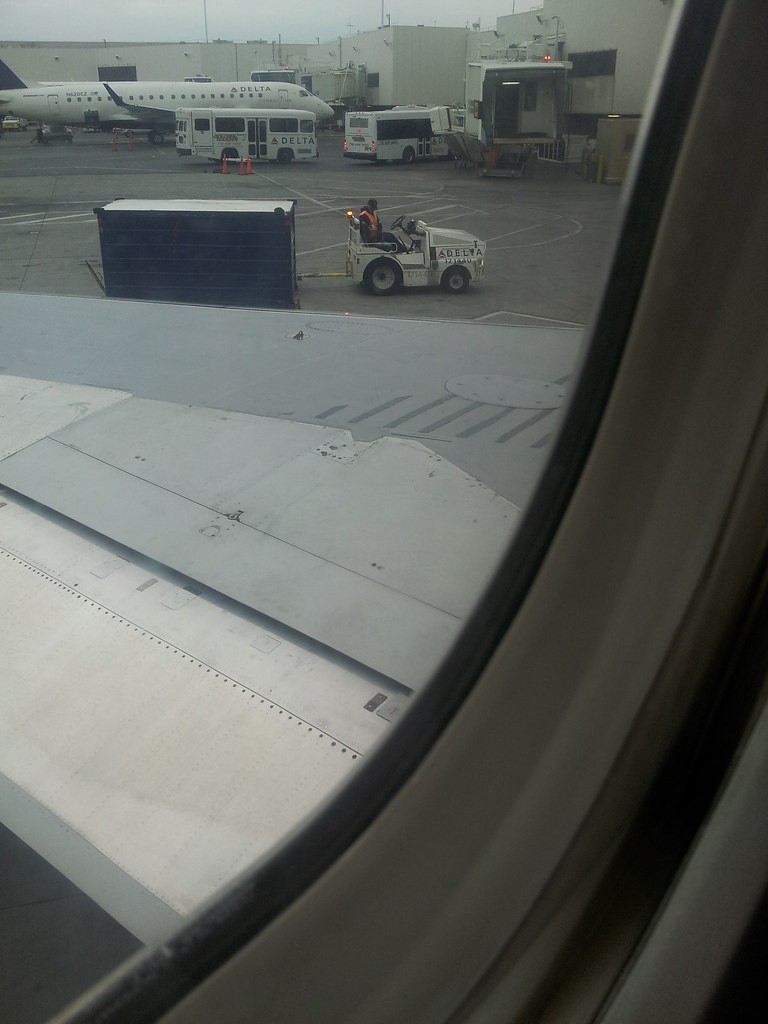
[112,136,117,152]
[246,159,255,174]
[128,133,136,151]
[238,160,247,175]
[222,153,231,174]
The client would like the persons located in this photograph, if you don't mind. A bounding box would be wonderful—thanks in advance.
[359,198,405,251]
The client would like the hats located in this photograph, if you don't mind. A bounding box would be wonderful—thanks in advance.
[368,199,378,210]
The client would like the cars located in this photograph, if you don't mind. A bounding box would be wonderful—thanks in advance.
[2,116,19,128]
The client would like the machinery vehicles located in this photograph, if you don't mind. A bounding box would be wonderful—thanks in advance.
[345,214,486,296]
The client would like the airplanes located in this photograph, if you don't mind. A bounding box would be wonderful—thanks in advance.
[0,59,335,145]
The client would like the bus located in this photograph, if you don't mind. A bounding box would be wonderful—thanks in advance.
[343,105,466,166]
[174,107,319,165]
